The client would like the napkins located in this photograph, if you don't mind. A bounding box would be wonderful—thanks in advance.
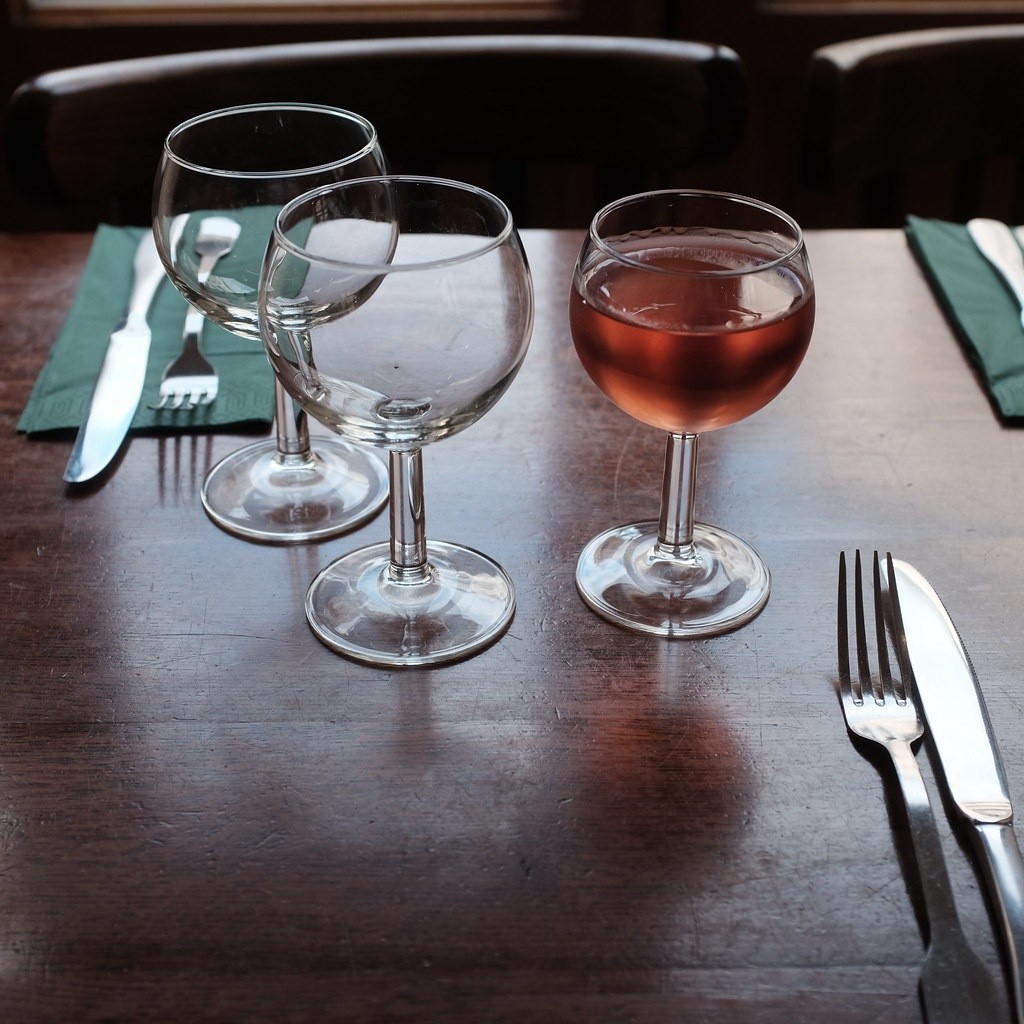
[14,201,316,443]
[902,213,1024,427]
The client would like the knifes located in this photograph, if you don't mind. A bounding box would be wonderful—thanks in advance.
[877,555,1024,1024]
[61,205,192,484]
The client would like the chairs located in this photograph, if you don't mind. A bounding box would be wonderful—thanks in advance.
[10,36,758,234]
[798,24,1024,230]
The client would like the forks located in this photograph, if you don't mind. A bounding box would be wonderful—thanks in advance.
[146,216,244,416]
[830,549,1009,1024]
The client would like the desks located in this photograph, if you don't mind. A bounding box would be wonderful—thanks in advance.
[0,225,1024,1024]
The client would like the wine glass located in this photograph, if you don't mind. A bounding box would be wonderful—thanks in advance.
[569,189,817,635]
[258,178,532,667]
[146,103,398,547]
